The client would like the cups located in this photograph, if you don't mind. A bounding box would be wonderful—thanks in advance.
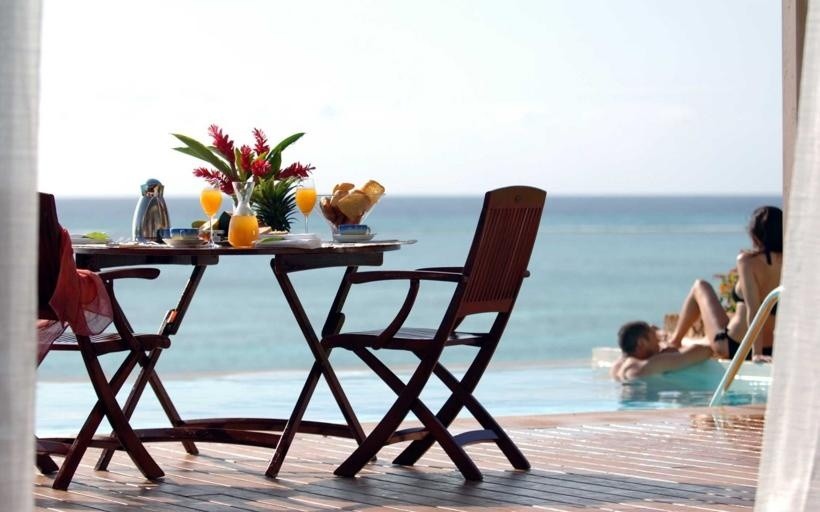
[338,224,371,235]
[170,227,200,239]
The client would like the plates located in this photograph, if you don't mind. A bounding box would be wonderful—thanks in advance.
[69,235,112,244]
[334,233,374,243]
[161,237,205,248]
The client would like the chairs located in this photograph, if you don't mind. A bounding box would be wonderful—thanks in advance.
[37,191,201,480]
[312,186,549,487]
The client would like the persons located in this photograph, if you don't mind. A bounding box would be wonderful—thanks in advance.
[608,320,715,389]
[653,200,787,366]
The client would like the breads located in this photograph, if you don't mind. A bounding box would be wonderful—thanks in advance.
[320,180,385,228]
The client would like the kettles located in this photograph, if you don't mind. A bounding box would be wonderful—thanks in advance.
[130,178,171,244]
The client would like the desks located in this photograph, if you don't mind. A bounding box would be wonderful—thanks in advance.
[70,230,419,480]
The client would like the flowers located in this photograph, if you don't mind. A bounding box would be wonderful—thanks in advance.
[170,119,318,233]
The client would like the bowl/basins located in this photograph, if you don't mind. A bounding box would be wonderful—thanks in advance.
[312,193,386,235]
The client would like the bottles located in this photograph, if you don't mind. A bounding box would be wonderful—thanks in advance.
[228,182,260,248]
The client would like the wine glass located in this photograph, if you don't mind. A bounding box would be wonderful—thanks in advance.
[198,179,223,249]
[295,176,317,234]
